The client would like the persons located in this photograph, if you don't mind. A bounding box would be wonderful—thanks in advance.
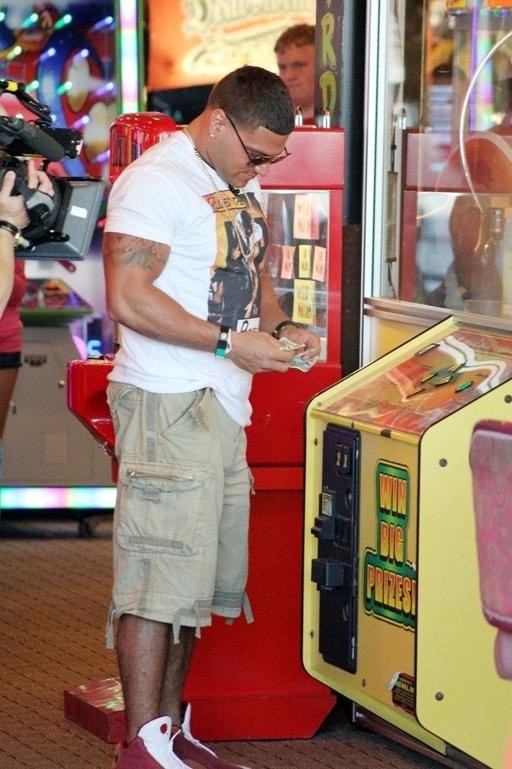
[98,62,324,768]
[431,77,511,324]
[211,237,252,331]
[227,211,265,318]
[1,154,59,320]
[270,22,319,128]
[1,253,26,444]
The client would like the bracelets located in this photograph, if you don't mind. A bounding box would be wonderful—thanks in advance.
[216,324,234,359]
[0,220,22,244]
[271,318,300,340]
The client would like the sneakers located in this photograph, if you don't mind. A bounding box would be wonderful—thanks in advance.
[173,703,248,769]
[112,715,188,767]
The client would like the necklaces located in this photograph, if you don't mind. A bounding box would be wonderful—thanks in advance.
[180,124,257,261]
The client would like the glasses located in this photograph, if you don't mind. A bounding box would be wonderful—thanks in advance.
[225,114,291,168]
